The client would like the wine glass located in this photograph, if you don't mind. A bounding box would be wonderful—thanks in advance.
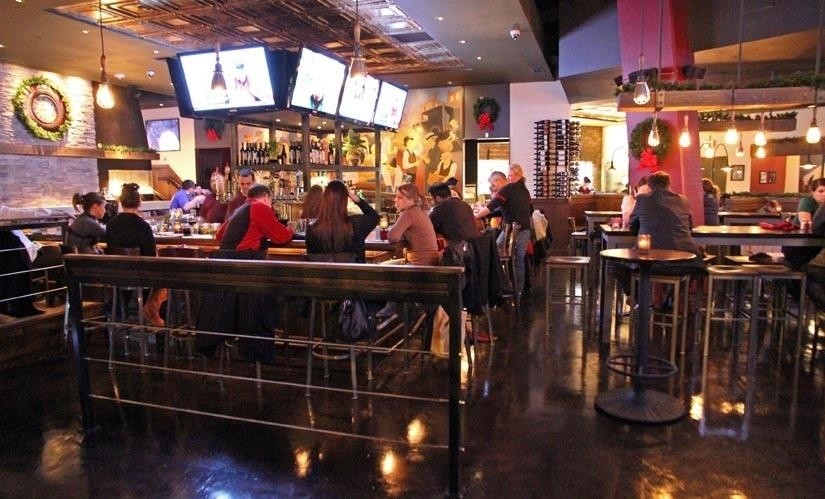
[186,219,199,235]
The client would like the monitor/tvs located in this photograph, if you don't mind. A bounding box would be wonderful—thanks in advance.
[371,80,408,132]
[284,47,348,120]
[176,46,279,113]
[145,118,180,151]
[339,67,382,128]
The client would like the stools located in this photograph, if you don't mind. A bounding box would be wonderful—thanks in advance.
[724,254,784,304]
[546,257,592,338]
[703,264,760,357]
[571,231,596,282]
[630,274,689,362]
[743,264,806,358]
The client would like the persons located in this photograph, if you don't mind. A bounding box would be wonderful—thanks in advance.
[427,182,479,267]
[785,178,825,298]
[194,184,204,196]
[577,177,591,194]
[701,177,718,227]
[301,185,324,219]
[61,191,107,254]
[711,185,722,201]
[169,180,205,215]
[226,169,255,223]
[804,202,825,311]
[216,185,296,251]
[469,171,532,300]
[105,184,169,326]
[444,177,462,199]
[506,164,535,296]
[304,180,381,263]
[197,172,228,223]
[375,185,440,317]
[630,171,704,311]
[621,175,652,228]
[757,200,781,214]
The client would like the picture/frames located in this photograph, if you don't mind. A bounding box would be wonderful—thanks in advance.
[767,171,776,183]
[731,165,744,181]
[760,171,768,183]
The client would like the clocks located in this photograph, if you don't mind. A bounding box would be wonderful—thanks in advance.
[25,85,67,130]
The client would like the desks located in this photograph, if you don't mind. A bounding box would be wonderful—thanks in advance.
[601,222,825,250]
[595,245,696,424]
[585,210,798,254]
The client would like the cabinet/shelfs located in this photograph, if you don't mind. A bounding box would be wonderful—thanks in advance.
[534,120,581,199]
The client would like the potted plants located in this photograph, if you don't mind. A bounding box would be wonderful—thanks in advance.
[614,66,825,112]
[750,135,825,158]
[697,112,798,131]
[725,191,810,212]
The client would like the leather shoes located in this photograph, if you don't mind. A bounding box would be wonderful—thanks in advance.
[376,302,399,317]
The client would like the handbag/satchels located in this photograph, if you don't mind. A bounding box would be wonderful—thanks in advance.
[332,298,375,343]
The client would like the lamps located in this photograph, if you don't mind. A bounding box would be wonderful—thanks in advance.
[212,0,226,90]
[725,83,740,145]
[706,130,715,159]
[700,142,714,158]
[736,0,745,157]
[806,0,825,144]
[634,1,650,105]
[96,0,116,108]
[754,111,767,146]
[755,146,767,159]
[678,112,691,148]
[712,144,732,183]
[351,1,367,77]
[648,0,663,147]
[607,145,628,170]
[799,154,816,170]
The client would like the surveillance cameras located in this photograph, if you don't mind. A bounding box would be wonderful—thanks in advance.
[509,29,521,41]
[147,70,155,79]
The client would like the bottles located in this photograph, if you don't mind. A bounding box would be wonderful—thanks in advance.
[533,119,569,200]
[278,173,285,197]
[375,226,380,241]
[380,229,388,240]
[308,139,336,165]
[223,162,230,180]
[295,176,301,202]
[347,176,353,186]
[238,138,302,166]
[210,167,221,194]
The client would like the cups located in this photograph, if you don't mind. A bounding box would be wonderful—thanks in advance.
[638,234,651,257]
[173,222,181,234]
[182,224,191,237]
[297,219,305,236]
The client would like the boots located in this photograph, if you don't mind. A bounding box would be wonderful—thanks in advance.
[141,284,169,327]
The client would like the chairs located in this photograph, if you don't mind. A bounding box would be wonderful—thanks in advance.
[60,244,107,352]
[208,249,294,389]
[404,240,474,371]
[158,247,209,375]
[304,253,374,398]
[498,222,520,278]
[461,227,513,344]
[104,247,161,372]
[498,228,530,317]
